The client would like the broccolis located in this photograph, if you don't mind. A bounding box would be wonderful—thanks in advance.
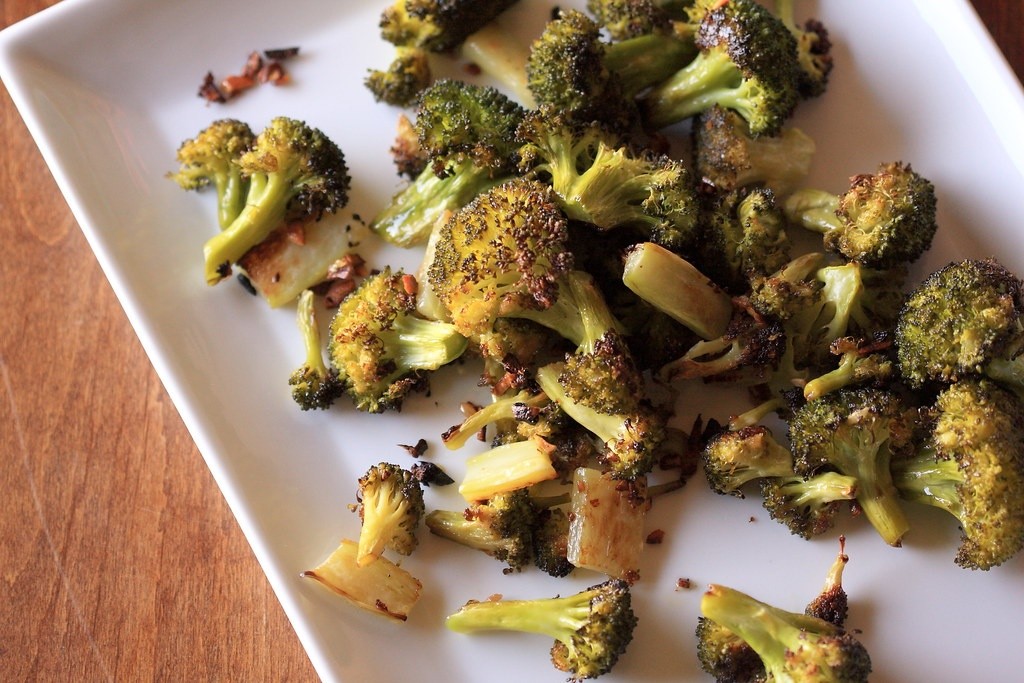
[169,0,1024,683]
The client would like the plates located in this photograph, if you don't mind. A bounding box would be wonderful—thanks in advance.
[0,0,1024,683]
